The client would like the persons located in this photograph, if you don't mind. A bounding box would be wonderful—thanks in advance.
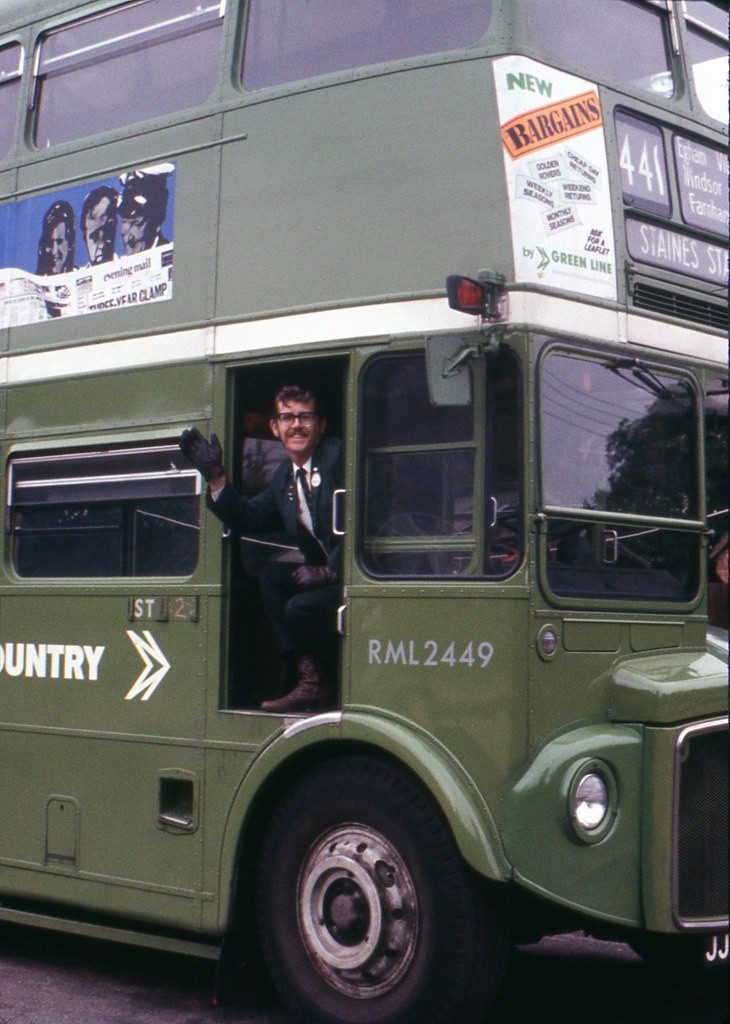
[179,383,370,711]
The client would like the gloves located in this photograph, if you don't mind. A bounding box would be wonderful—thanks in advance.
[291,565,337,588]
[179,428,224,482]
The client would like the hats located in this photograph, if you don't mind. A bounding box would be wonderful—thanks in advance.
[115,162,174,219]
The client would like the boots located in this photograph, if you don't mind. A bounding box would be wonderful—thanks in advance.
[261,655,332,711]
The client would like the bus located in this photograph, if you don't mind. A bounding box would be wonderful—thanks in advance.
[2,0,729,1023]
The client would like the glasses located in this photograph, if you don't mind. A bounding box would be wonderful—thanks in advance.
[276,412,318,426]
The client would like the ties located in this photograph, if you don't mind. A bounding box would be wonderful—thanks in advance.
[296,469,316,530]
